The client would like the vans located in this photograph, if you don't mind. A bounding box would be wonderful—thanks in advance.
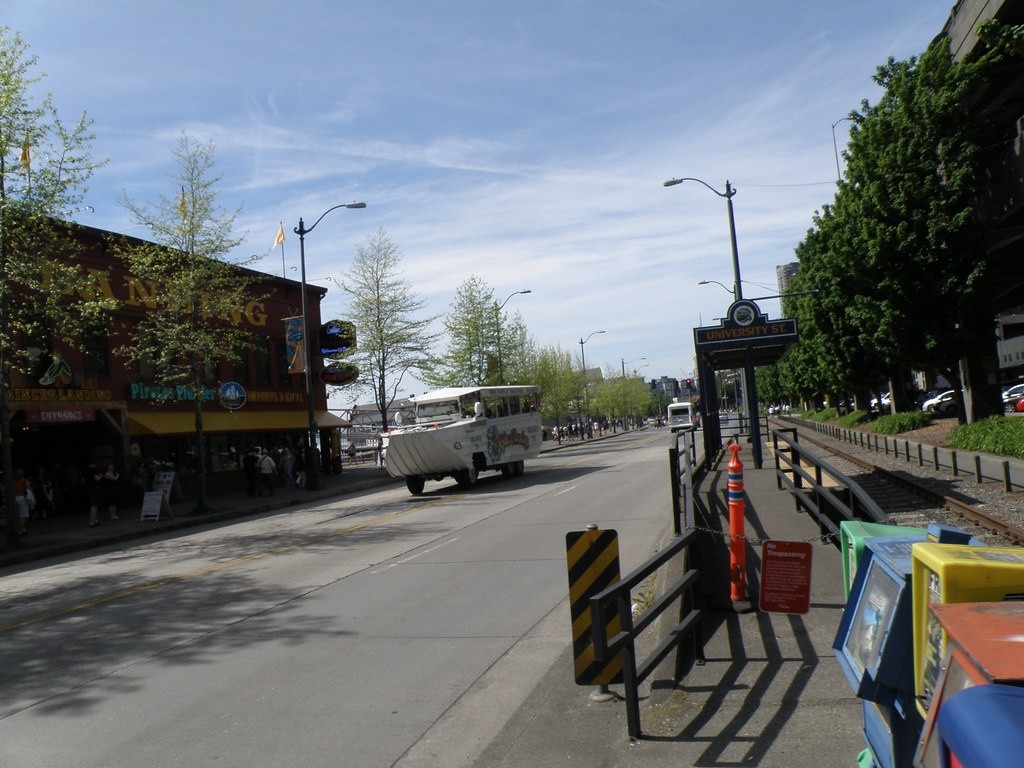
[666,401,698,433]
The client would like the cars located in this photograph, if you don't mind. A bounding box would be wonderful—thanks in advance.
[840,382,1024,418]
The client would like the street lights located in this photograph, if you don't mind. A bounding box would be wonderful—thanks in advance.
[622,357,646,431]
[494,289,532,385]
[579,330,606,439]
[292,201,367,494]
[661,177,743,301]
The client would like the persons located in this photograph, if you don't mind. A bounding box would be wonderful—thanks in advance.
[542,415,661,441]
[347,443,358,465]
[137,461,148,513]
[486,402,531,418]
[13,462,119,535]
[769,404,789,418]
[448,405,467,417]
[245,443,322,498]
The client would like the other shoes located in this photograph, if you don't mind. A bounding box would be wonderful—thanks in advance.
[89,522,100,527]
[19,531,27,537]
[111,517,118,520]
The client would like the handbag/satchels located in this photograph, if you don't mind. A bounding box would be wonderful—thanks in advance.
[24,479,30,502]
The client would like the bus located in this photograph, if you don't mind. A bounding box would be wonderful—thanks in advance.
[383,383,544,498]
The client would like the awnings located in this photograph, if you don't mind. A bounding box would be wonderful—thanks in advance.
[7,401,126,433]
[127,410,352,435]
